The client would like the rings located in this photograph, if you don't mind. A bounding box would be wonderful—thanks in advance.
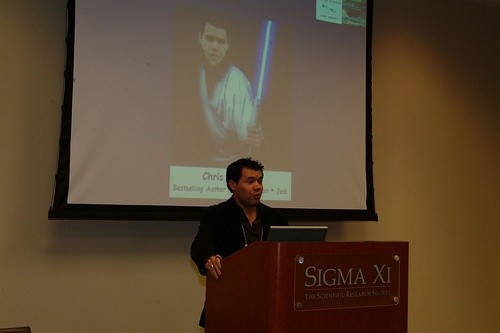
[210,264,214,268]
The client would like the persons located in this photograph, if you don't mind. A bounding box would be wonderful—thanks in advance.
[162,15,263,170]
[189,158,291,333]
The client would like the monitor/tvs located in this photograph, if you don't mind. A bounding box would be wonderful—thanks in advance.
[267,226,329,241]
